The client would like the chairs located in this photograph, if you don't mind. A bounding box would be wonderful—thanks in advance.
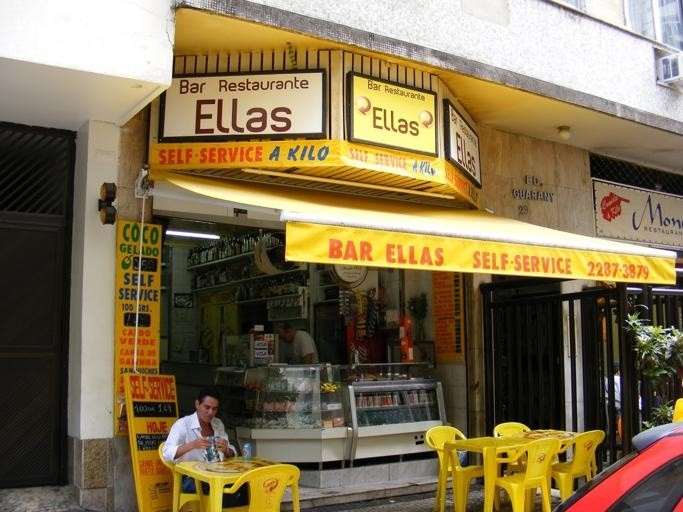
[201,462,301,512]
[420,416,608,511]
[157,440,205,512]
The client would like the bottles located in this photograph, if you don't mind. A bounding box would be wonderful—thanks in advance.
[354,389,434,409]
[186,234,257,267]
[241,440,251,459]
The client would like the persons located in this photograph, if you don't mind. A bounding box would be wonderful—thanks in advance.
[276,320,319,365]
[160,388,248,508]
[604,362,643,440]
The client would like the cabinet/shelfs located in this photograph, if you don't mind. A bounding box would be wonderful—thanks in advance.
[311,266,380,304]
[182,235,312,311]
[158,354,354,473]
[338,371,449,468]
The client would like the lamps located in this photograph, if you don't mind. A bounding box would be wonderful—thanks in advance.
[556,126,571,142]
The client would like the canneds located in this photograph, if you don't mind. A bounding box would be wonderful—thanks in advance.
[242,443,252,460]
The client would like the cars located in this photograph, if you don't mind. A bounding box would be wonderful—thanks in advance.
[550,422,683,511]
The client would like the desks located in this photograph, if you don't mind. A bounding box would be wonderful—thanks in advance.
[172,454,287,512]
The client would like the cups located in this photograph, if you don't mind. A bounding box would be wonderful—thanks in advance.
[247,287,255,300]
